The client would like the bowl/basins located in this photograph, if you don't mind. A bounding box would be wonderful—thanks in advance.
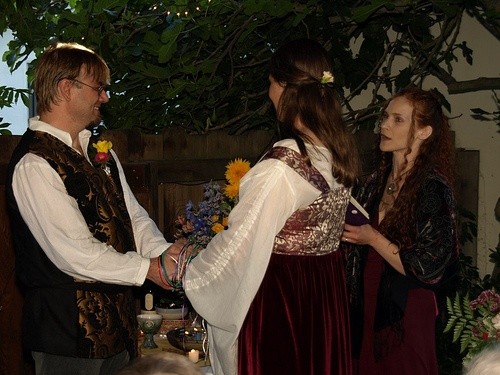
[156,308,188,320]
[167,327,208,352]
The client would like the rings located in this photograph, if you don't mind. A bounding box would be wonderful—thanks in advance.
[346,238,347,241]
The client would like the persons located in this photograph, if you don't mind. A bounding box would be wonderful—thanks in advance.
[5,42,206,375]
[180,38,362,375]
[340,88,462,375]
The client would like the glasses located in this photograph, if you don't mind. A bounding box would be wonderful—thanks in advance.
[68,77,107,96]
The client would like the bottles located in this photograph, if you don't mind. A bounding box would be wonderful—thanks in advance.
[143,281,157,311]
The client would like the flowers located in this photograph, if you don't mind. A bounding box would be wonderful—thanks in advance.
[320,70,335,84]
[173,157,254,288]
[91,139,113,163]
[442,284,500,354]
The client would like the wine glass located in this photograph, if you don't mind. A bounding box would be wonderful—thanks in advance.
[137,314,163,349]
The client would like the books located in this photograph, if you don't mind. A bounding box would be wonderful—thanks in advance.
[345,196,370,226]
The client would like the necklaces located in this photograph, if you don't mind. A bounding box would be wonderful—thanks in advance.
[385,171,409,194]
